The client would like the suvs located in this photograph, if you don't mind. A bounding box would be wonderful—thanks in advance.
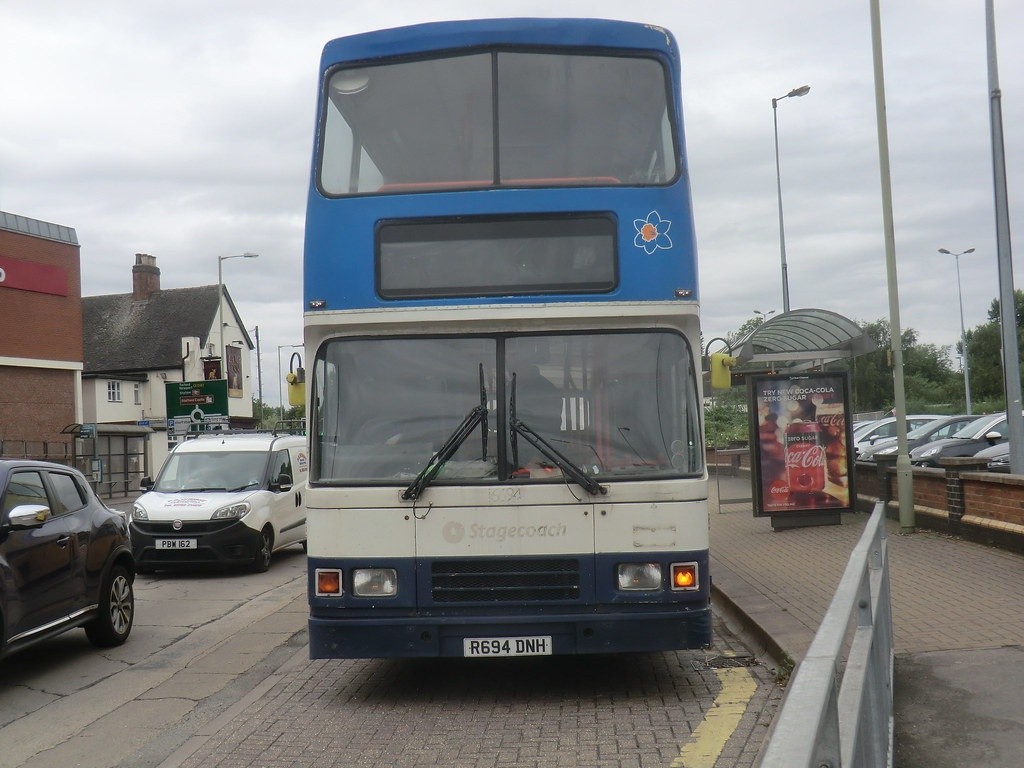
[0,460,136,659]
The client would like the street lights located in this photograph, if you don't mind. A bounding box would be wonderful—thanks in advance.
[938,247,978,415]
[219,253,260,378]
[753,309,776,368]
[772,84,811,315]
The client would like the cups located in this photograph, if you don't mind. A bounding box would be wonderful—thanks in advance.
[815,403,848,487]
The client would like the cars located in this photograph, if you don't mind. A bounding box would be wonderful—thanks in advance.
[855,415,971,457]
[973,442,1011,474]
[856,413,986,463]
[909,410,1024,467]
[853,420,890,440]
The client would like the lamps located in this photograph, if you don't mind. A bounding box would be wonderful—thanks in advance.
[233,341,244,345]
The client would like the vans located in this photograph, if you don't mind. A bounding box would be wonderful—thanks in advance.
[129,431,309,572]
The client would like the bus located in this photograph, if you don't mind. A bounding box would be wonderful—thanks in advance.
[286,17,735,661]
